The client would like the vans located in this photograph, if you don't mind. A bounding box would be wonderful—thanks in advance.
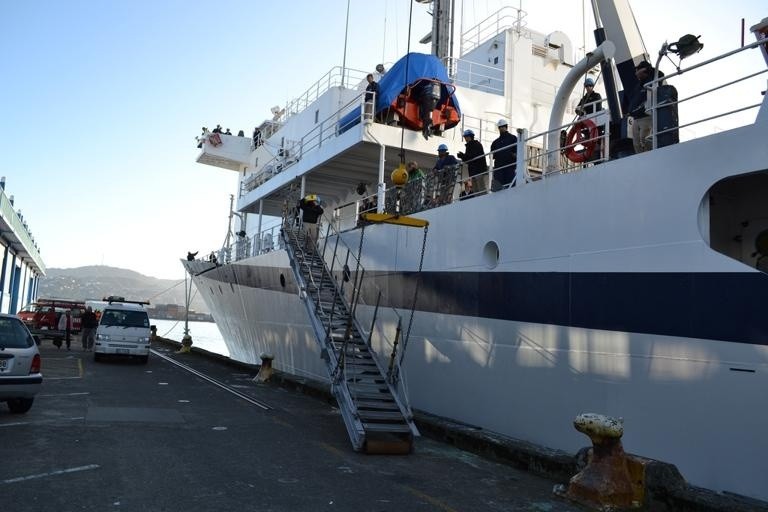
[93,296,154,365]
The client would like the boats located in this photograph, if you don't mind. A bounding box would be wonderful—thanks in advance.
[182,0,766,509]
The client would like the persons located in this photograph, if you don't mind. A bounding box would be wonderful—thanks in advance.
[459,179,474,201]
[408,161,425,181]
[187,251,198,262]
[433,144,458,204]
[628,68,652,153]
[457,129,488,197]
[365,74,377,101]
[202,125,261,149]
[210,251,216,263]
[489,120,517,192]
[300,198,324,255]
[81,306,96,352]
[58,308,73,351]
[575,78,602,167]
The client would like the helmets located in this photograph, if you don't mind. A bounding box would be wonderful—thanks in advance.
[316,196,320,204]
[463,130,474,137]
[438,144,448,151]
[585,78,594,87]
[498,120,507,126]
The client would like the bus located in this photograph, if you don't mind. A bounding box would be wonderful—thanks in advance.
[17,297,88,333]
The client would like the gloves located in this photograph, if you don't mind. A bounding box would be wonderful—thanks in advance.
[457,151,463,158]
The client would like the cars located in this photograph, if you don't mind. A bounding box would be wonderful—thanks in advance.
[0,310,43,416]
